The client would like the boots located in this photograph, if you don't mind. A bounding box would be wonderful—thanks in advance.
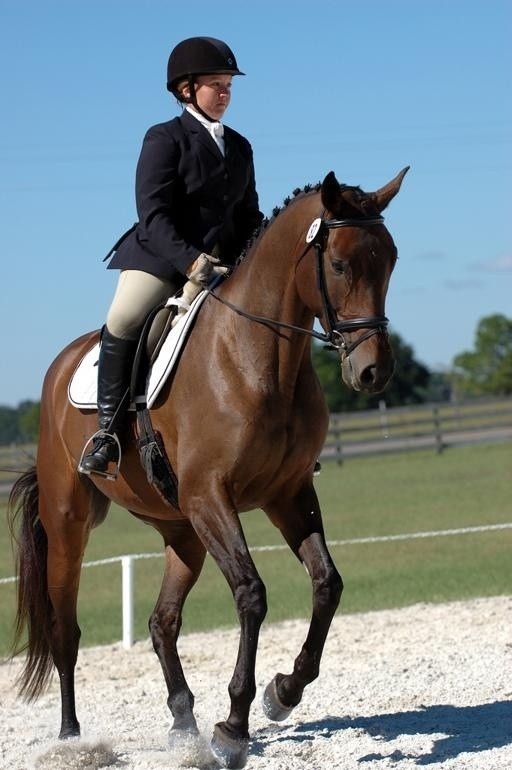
[82,323,138,473]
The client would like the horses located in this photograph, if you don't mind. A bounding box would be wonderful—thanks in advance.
[0,163,413,765]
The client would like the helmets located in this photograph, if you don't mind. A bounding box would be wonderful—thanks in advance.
[166,36,246,92]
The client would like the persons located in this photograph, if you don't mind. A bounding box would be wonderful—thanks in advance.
[76,34,321,479]
